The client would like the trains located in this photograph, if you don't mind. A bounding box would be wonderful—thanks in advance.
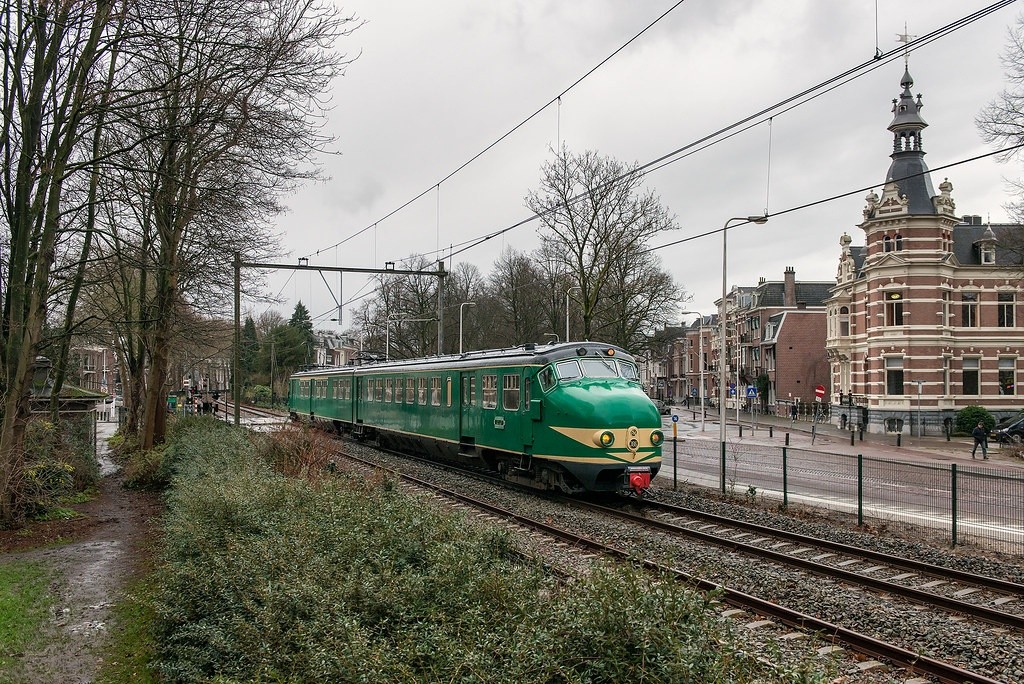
[287,339,665,504]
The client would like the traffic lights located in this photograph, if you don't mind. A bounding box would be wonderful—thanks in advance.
[211,389,221,400]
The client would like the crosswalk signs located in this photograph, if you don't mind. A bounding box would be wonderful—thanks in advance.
[747,387,757,398]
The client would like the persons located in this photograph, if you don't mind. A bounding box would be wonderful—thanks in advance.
[680,394,688,407]
[972,420,989,459]
[791,401,798,423]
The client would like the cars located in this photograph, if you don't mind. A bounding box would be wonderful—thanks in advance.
[650,399,671,415]
[990,408,1024,444]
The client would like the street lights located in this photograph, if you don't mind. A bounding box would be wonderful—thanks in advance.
[682,311,705,432]
[458,302,477,355]
[565,285,594,342]
[361,324,377,357]
[386,313,408,361]
[727,327,740,423]
[719,214,769,490]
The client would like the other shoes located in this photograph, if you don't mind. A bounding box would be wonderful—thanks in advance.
[983,456,989,459]
[972,454,975,459]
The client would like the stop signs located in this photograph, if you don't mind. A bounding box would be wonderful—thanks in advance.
[815,385,825,397]
